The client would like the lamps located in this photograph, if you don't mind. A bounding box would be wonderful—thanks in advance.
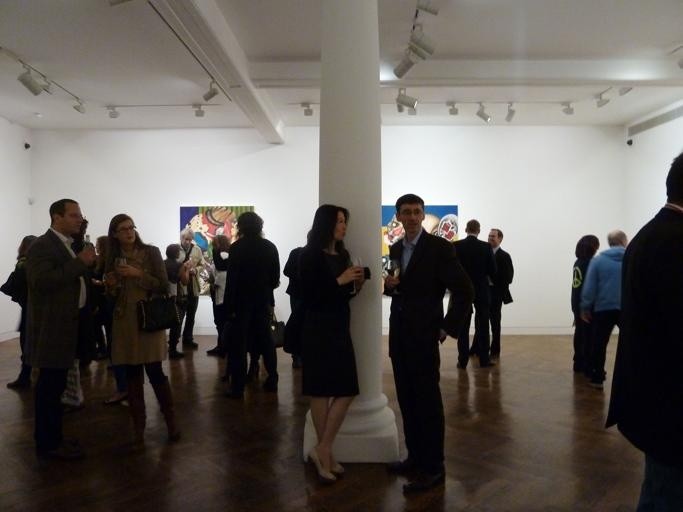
[103,103,219,119]
[301,102,314,117]
[390,1,440,80]
[202,78,220,102]
[561,99,576,116]
[445,100,517,124]
[594,92,610,108]
[17,60,87,115]
[393,88,419,113]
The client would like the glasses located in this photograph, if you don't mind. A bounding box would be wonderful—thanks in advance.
[112,226,136,232]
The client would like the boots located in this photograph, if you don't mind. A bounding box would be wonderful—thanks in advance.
[125,382,146,455]
[150,376,180,443]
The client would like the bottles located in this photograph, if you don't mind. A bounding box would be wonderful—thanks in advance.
[84,235,92,250]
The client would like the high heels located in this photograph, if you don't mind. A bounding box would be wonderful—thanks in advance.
[305,449,345,484]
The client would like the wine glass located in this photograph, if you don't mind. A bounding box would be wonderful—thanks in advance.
[115,258,127,287]
[385,260,400,294]
[350,257,362,294]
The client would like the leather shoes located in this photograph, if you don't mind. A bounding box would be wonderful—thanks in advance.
[403,471,445,492]
[457,346,498,369]
[388,461,408,473]
[574,364,606,389]
[168,342,278,400]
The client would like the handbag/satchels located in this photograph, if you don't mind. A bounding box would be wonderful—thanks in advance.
[0,271,16,296]
[137,275,181,332]
[267,312,285,347]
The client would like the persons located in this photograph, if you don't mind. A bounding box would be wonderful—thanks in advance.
[604,150,682,511]
[285,228,312,368]
[382,193,476,494]
[580,231,629,389]
[291,204,366,482]
[469,227,514,355]
[1,197,280,462]
[451,221,497,368]
[572,234,607,375]
